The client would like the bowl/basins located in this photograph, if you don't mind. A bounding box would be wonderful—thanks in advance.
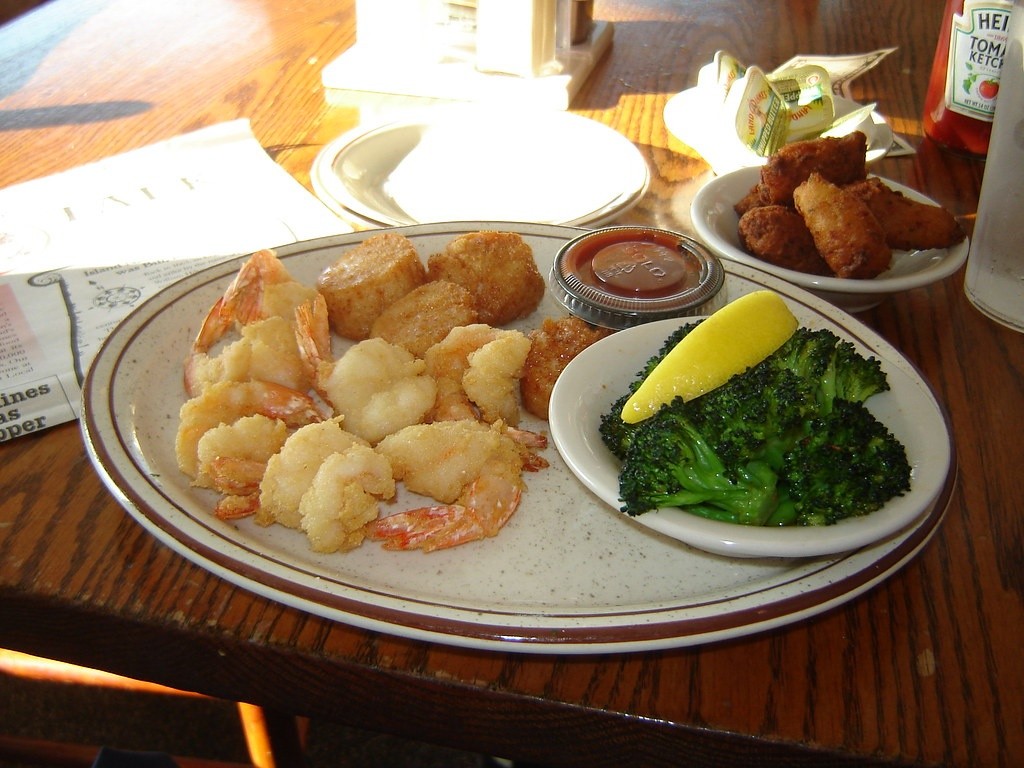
[549,224,726,335]
[692,163,969,313]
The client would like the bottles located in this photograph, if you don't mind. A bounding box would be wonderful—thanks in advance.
[964,0,1024,333]
[923,0,1015,160]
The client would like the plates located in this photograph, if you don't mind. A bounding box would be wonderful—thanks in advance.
[549,315,950,560]
[309,110,650,228]
[78,220,958,655]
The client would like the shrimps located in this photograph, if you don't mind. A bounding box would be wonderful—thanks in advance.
[163,232,551,554]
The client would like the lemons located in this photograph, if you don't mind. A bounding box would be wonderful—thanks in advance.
[621,291,799,424]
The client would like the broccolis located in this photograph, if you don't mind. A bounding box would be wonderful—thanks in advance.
[599,315,913,530]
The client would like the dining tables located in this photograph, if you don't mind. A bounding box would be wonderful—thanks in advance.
[1,0,1024,768]
